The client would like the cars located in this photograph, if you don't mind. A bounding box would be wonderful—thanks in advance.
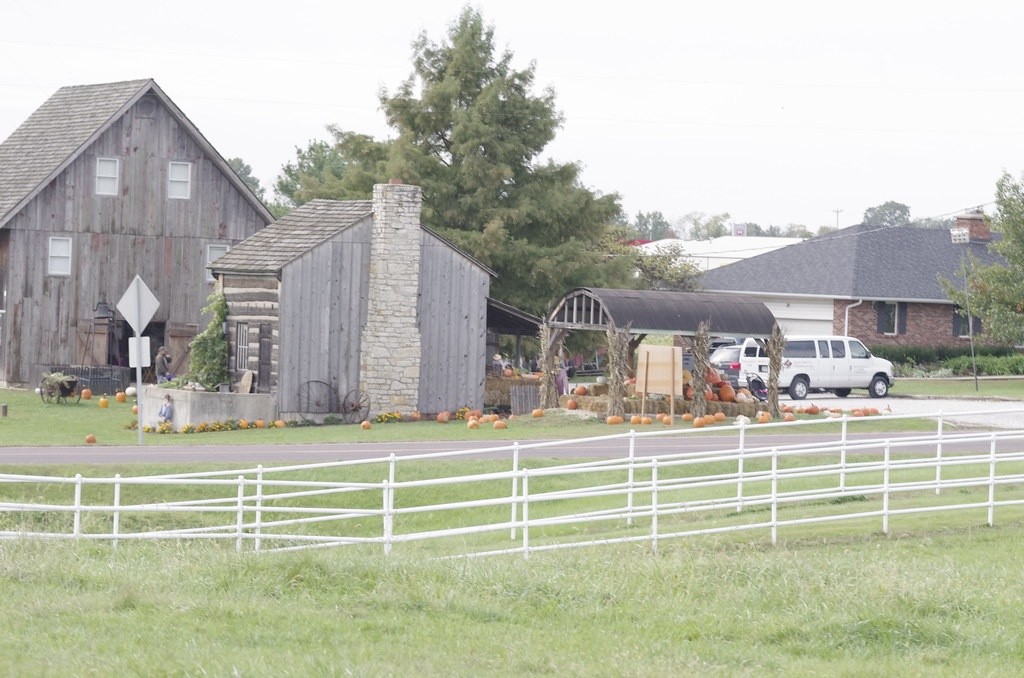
[708,343,743,391]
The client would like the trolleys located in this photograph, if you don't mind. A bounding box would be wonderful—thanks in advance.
[39,373,83,405]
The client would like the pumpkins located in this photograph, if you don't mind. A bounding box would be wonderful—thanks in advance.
[361,419,373,429]
[568,396,579,409]
[86,433,97,443]
[533,404,543,418]
[99,396,109,408]
[505,364,515,378]
[241,416,284,429]
[133,398,139,414]
[683,369,756,401]
[756,403,879,421]
[116,389,125,401]
[82,389,93,399]
[575,385,588,396]
[439,410,520,429]
[607,413,729,429]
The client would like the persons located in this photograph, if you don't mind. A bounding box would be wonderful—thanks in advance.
[493,354,505,370]
[155,347,172,385]
[157,394,173,425]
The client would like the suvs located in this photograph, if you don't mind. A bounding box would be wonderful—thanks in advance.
[737,334,896,398]
